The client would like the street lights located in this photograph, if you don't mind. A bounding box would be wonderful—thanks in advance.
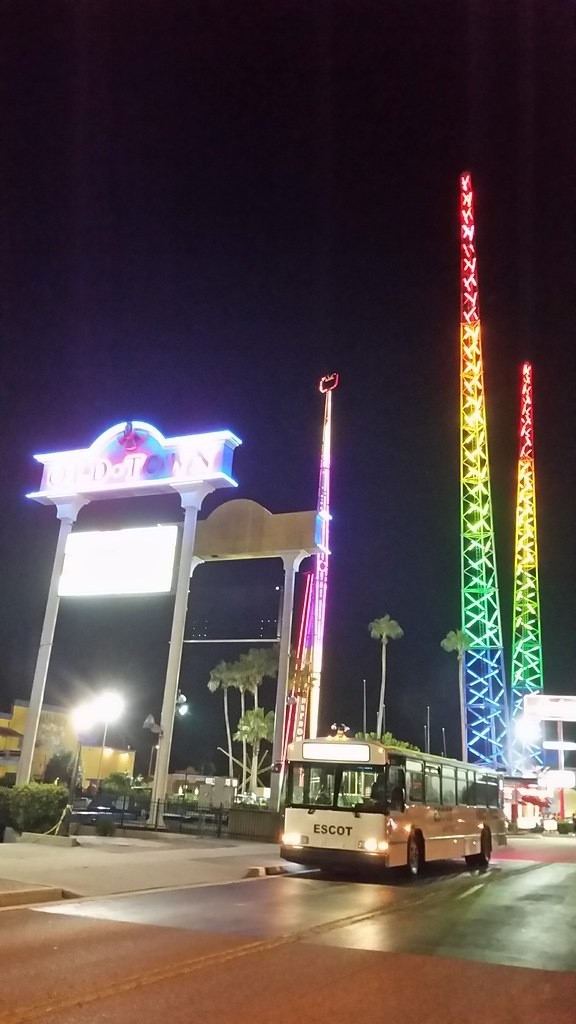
[67,692,124,804]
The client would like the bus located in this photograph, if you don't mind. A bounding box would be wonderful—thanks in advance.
[272,738,502,877]
